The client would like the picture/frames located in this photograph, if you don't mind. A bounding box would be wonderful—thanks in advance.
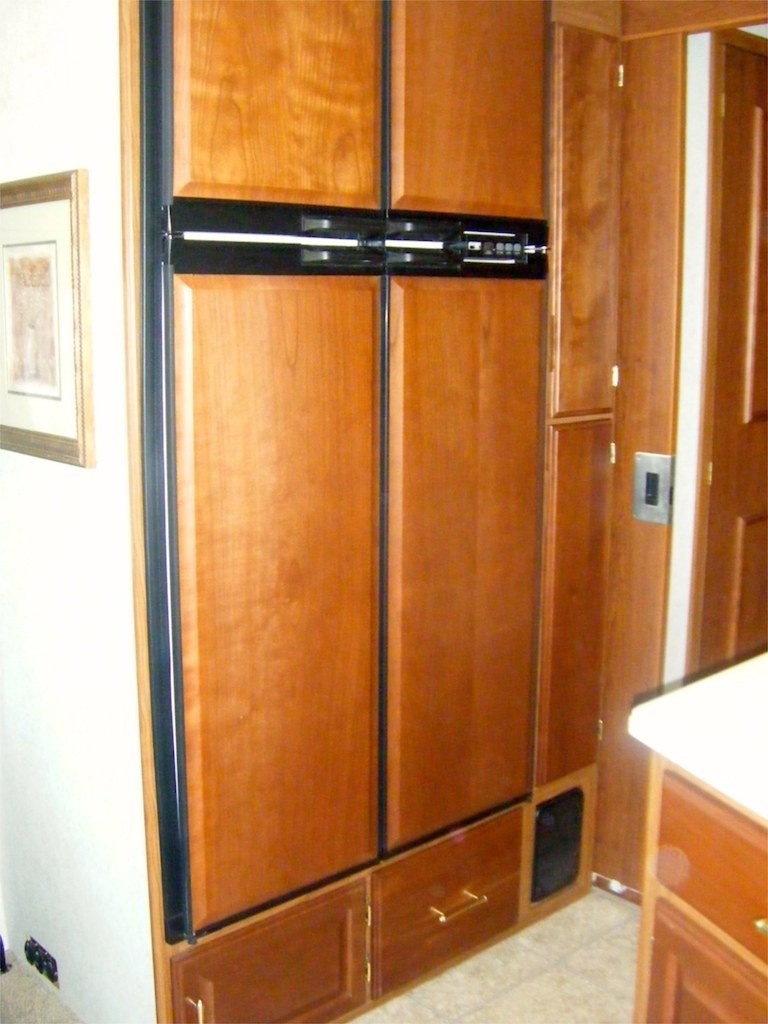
[0,168,94,469]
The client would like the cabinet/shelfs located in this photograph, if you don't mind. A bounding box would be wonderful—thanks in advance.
[116,2,631,1024]
[632,755,768,1024]
